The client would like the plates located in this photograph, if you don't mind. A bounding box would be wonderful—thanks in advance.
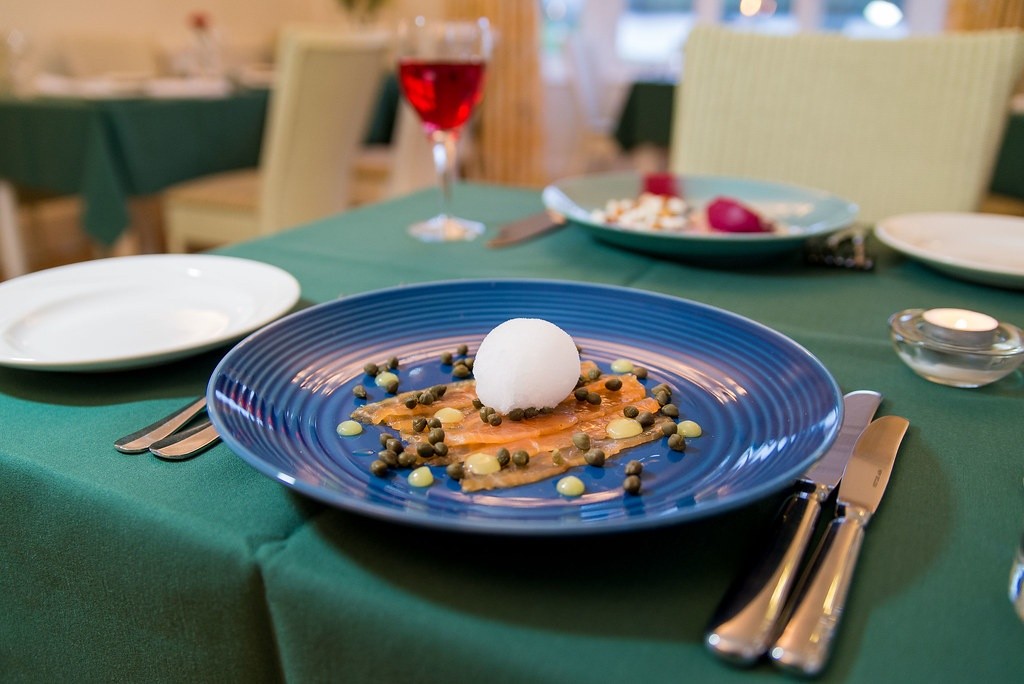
[541,169,861,260]
[0,253,302,374]
[871,212,1024,289]
[206,277,847,539]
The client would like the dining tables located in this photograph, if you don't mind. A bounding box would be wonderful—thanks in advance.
[0,178,1022,684]
[1,69,398,278]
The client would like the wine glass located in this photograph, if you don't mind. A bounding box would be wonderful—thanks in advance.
[395,17,495,243]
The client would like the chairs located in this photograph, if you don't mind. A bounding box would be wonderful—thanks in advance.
[670,22,1022,218]
[156,28,395,255]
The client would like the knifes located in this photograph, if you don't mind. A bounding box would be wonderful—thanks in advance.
[769,415,910,678]
[701,390,884,668]
